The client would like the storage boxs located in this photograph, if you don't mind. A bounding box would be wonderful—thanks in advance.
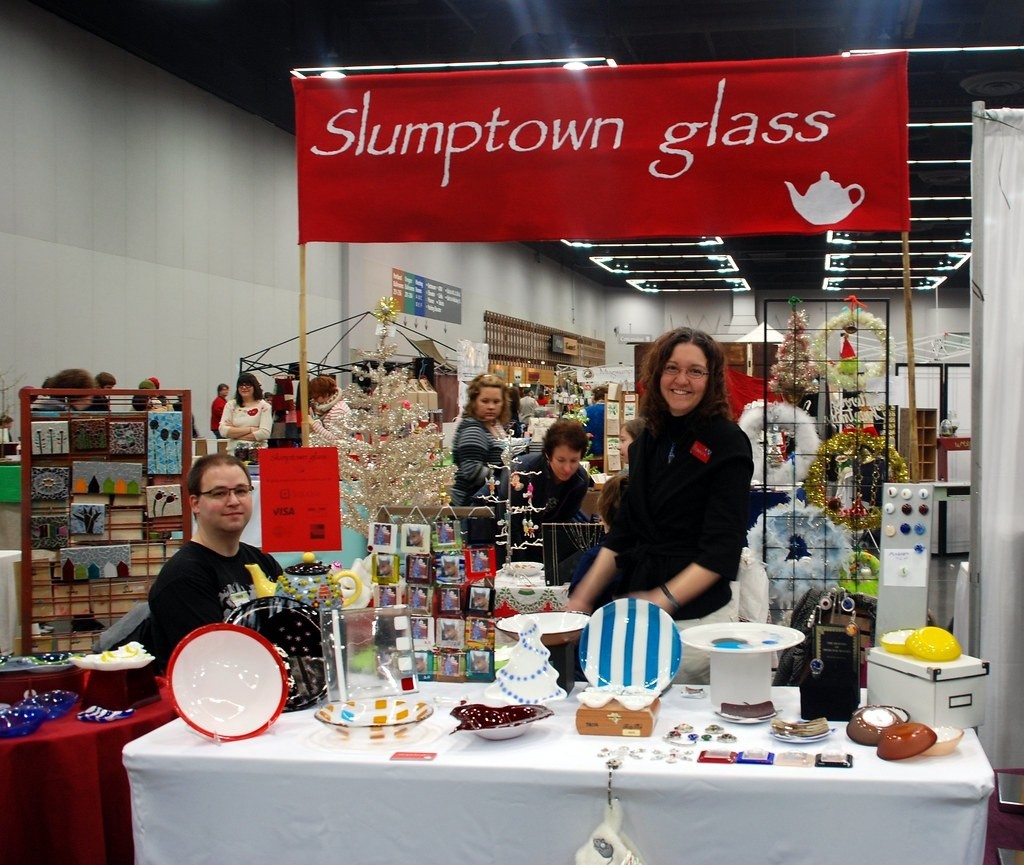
[866,647,993,733]
[0,407,189,655]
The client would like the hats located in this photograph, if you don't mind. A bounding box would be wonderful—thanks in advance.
[139,377,159,389]
[524,388,531,394]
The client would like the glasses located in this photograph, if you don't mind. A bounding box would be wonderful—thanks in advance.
[201,484,254,498]
[662,364,710,380]
[239,384,251,388]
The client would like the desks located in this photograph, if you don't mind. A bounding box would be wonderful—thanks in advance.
[0,669,175,865]
[936,436,971,482]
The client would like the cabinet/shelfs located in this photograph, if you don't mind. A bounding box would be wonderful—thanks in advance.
[898,407,938,481]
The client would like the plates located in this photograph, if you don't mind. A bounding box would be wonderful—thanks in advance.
[314,700,433,743]
[502,562,544,576]
[67,654,155,671]
[493,611,592,646]
[166,596,334,743]
[768,718,838,743]
[578,598,682,691]
[715,700,778,723]
[0,651,86,673]
[680,623,806,653]
[448,704,555,740]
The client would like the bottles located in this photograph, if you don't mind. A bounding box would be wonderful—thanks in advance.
[501,556,516,582]
[940,418,953,437]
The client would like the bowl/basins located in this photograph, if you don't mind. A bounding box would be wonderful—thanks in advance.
[846,707,904,747]
[878,629,916,655]
[904,626,962,662]
[853,705,910,724]
[921,726,965,756]
[877,723,937,760]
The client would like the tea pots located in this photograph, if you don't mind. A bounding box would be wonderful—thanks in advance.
[244,552,362,611]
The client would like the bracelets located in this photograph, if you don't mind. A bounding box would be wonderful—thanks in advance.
[660,583,680,610]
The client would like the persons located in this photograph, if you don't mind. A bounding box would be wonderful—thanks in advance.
[148,453,283,675]
[451,374,647,584]
[17,369,354,457]
[563,326,755,684]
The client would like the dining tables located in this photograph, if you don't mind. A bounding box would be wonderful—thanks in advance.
[120,679,996,865]
